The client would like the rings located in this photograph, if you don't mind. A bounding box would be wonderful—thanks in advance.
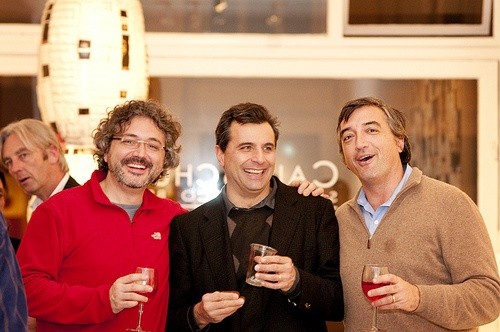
[393,296,396,302]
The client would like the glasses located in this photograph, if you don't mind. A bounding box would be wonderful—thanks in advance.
[110,134,165,151]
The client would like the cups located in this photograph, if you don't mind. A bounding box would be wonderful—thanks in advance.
[245,243,277,287]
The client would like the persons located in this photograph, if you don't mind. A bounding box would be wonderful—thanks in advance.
[293,96,500,332]
[0,174,29,332]
[0,119,82,204]
[165,101,345,332]
[16,99,324,332]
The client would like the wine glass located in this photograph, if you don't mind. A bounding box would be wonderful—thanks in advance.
[126,267,155,332]
[362,264,389,332]
